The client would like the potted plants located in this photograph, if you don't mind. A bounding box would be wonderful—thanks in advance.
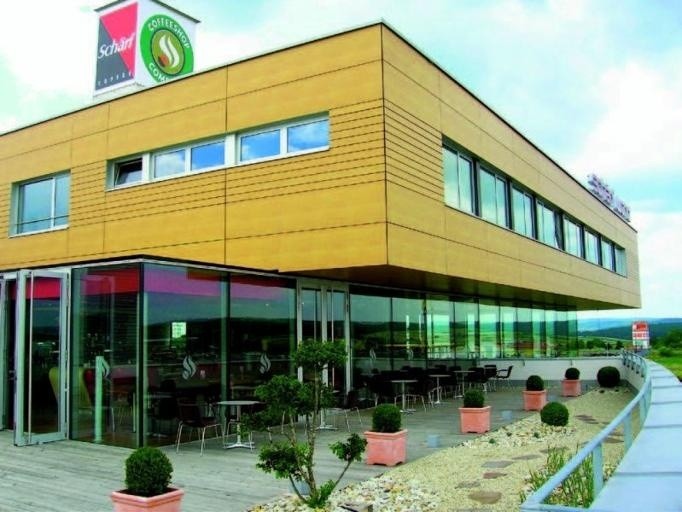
[110,447,185,512]
[364,404,408,466]
[522,375,549,412]
[560,367,582,396]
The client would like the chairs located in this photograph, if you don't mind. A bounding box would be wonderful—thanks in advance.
[117,364,514,457]
